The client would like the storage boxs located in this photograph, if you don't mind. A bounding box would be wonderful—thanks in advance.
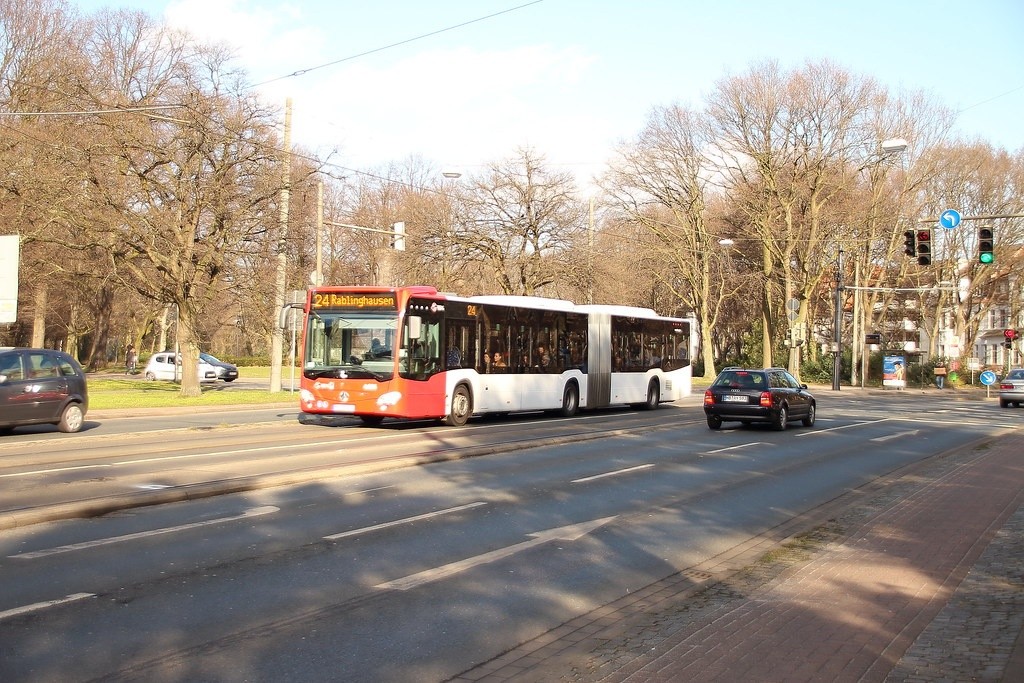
[934,368,946,375]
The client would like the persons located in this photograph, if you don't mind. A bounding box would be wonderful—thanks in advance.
[935,361,946,389]
[483,352,491,363]
[126,342,134,366]
[538,345,556,366]
[494,352,506,366]
[523,354,529,364]
[125,348,136,375]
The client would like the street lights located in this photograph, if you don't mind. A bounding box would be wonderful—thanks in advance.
[440,169,462,293]
[691,238,734,333]
[850,139,910,387]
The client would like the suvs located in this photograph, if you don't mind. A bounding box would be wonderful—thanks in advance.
[703,366,817,431]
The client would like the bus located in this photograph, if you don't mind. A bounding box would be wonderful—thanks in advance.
[279,282,693,427]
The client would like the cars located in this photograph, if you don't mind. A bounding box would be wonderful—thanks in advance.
[999,370,1024,408]
[143,352,219,385]
[0,347,89,433]
[200,352,239,382]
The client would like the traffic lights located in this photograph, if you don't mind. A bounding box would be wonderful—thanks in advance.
[1003,329,1014,337]
[903,230,917,258]
[916,227,933,266]
[976,226,994,265]
[1012,329,1019,341]
[791,338,804,348]
[390,221,406,251]
[1005,337,1012,349]
